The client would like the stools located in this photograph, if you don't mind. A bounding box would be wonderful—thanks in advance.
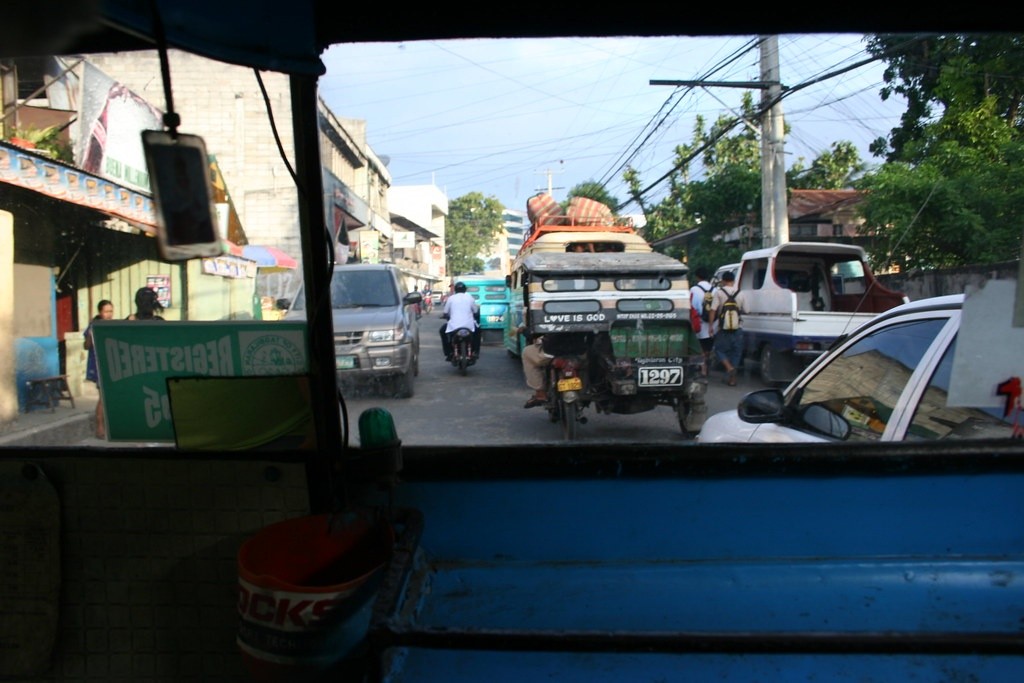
[25,375,76,413]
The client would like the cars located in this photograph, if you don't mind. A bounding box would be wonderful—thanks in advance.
[461,280,511,345]
[431,291,443,305]
[696,293,1024,444]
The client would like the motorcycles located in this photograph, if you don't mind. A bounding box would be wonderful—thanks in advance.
[439,315,479,377]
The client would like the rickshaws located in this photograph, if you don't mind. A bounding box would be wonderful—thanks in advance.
[521,252,709,442]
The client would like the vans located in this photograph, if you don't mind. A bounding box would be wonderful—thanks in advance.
[276,264,422,400]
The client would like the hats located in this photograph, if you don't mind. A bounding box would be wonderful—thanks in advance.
[135,286,158,300]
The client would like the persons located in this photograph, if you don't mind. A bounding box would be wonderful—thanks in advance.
[124,287,165,321]
[439,282,481,362]
[422,285,432,310]
[518,325,592,410]
[84,300,114,439]
[689,268,718,376]
[412,286,421,314]
[708,271,751,385]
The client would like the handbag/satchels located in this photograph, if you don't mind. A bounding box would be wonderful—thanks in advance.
[689,292,702,332]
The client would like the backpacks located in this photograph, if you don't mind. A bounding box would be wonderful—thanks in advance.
[718,286,742,331]
[694,282,719,323]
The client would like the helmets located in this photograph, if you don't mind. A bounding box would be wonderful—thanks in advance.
[453,282,467,293]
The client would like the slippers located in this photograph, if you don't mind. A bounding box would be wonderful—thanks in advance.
[524,394,550,407]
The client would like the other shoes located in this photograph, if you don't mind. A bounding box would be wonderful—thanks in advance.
[721,368,737,384]
[692,376,708,386]
[445,353,454,361]
[472,352,479,357]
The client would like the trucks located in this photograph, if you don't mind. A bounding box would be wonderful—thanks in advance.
[709,242,911,389]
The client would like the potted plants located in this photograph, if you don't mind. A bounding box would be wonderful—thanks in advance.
[10,126,59,160]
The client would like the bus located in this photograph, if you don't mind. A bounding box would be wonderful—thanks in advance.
[506,216,653,364]
